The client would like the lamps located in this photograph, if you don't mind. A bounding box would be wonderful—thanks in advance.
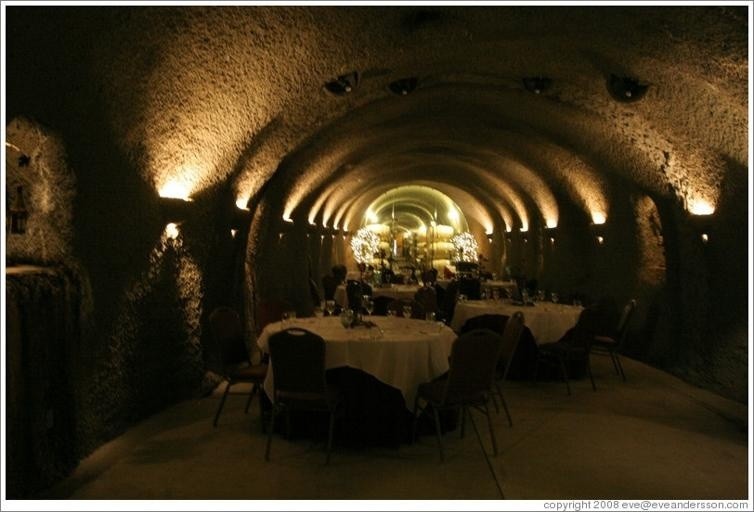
[325,71,417,98]
[524,77,649,103]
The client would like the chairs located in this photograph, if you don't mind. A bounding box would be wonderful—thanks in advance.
[411,330,499,463]
[455,312,526,428]
[308,275,634,397]
[207,306,270,425]
[267,328,351,463]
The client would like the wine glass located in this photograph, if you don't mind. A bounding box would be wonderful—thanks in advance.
[402,306,412,328]
[345,309,354,335]
[281,311,297,329]
[365,301,374,321]
[314,306,324,329]
[483,287,561,310]
[325,300,335,324]
[425,311,436,333]
[386,309,397,331]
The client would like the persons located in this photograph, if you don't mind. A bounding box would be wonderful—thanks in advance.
[327,261,446,319]
